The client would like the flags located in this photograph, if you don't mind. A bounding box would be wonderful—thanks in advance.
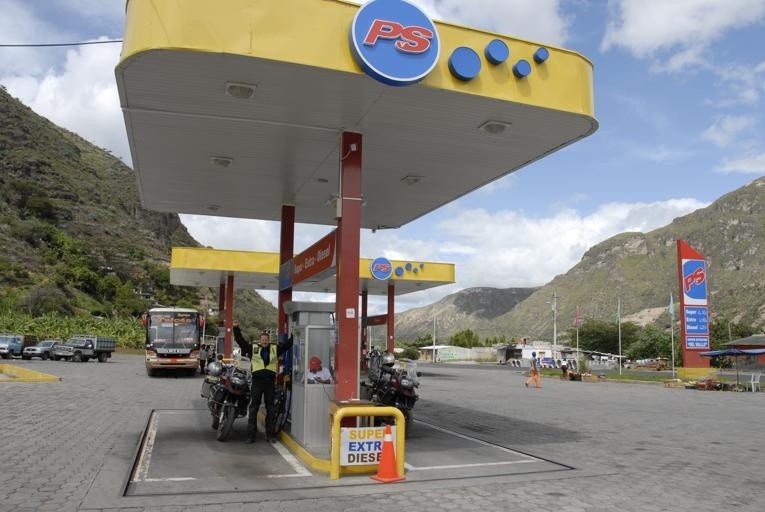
[615,301,622,325]
[669,293,675,321]
[576,305,582,325]
[552,292,559,317]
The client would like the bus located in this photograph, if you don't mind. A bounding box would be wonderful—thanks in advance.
[141,307,204,377]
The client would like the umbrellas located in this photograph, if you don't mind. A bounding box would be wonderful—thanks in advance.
[699,348,765,386]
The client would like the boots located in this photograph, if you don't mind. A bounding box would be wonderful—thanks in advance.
[244,407,258,444]
[263,407,276,443]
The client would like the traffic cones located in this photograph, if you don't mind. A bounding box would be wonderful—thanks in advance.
[370,424,404,483]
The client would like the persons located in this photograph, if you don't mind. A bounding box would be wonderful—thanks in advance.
[560,357,568,376]
[199,343,217,374]
[231,319,292,444]
[524,350,543,388]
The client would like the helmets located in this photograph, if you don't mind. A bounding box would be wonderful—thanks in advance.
[206,361,222,377]
[379,351,395,366]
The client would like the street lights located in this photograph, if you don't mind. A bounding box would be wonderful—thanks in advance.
[545,292,556,367]
[432,304,453,363]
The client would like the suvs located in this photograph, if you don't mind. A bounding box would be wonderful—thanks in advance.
[22,340,60,359]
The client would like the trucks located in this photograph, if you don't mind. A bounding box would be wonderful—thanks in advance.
[52,335,115,362]
[0,333,36,360]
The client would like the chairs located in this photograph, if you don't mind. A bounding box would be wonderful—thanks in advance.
[746,373,762,392]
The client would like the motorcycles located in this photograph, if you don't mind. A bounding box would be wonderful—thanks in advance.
[198,352,252,441]
[361,346,422,434]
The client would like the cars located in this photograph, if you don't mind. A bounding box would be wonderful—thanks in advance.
[622,361,638,370]
